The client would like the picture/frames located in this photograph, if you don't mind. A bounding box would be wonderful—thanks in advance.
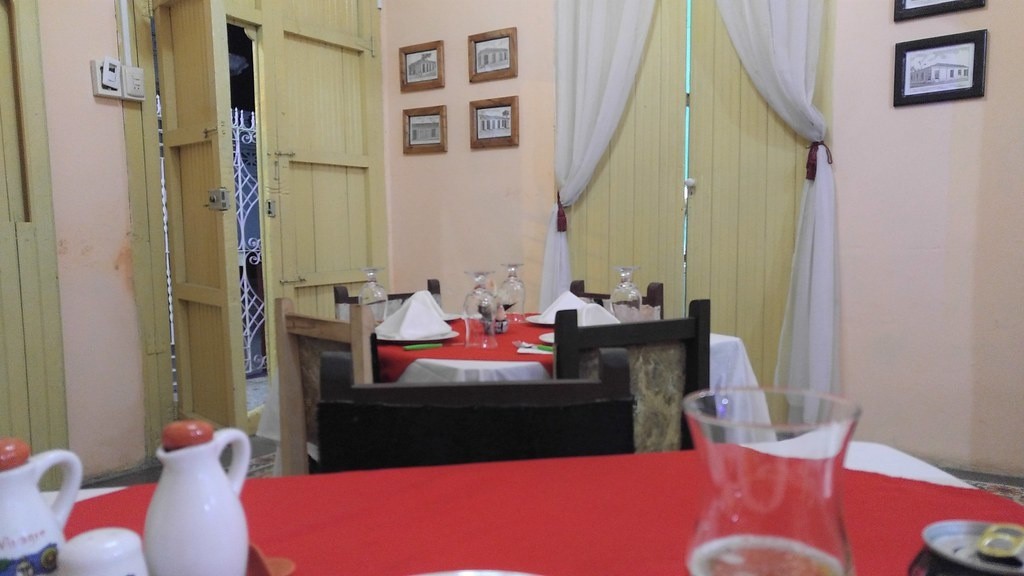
[403,105,447,154]
[468,27,518,82]
[399,40,445,93]
[893,0,985,21]
[469,96,519,149]
[893,28,988,107]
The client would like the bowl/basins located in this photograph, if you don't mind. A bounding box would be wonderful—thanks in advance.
[479,319,508,333]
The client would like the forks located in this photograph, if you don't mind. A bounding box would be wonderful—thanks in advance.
[512,341,553,351]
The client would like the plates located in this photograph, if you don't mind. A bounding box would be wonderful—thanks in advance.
[538,332,554,343]
[376,331,459,341]
[460,314,482,320]
[443,313,460,320]
[686,535,844,576]
[524,315,554,325]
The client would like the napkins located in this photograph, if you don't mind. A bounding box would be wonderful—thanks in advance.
[541,291,584,322]
[577,303,619,326]
[375,290,452,339]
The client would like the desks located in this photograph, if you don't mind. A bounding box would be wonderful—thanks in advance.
[376,313,777,449]
[64,431,1024,576]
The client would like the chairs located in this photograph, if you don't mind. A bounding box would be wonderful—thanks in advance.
[272,279,711,475]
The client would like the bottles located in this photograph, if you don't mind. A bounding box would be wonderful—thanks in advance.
[56,527,148,576]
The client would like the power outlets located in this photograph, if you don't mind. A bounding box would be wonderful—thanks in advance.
[121,66,145,101]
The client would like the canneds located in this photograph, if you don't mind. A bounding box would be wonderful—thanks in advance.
[907,519,1024,576]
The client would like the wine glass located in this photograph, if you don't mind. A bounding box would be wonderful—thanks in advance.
[498,263,527,323]
[610,266,641,322]
[358,266,388,327]
[463,270,498,348]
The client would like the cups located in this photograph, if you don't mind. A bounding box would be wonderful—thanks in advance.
[682,388,863,576]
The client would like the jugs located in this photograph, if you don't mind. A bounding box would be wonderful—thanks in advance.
[143,419,251,576]
[0,438,82,576]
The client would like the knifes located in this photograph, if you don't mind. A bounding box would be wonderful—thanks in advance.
[403,342,480,350]
